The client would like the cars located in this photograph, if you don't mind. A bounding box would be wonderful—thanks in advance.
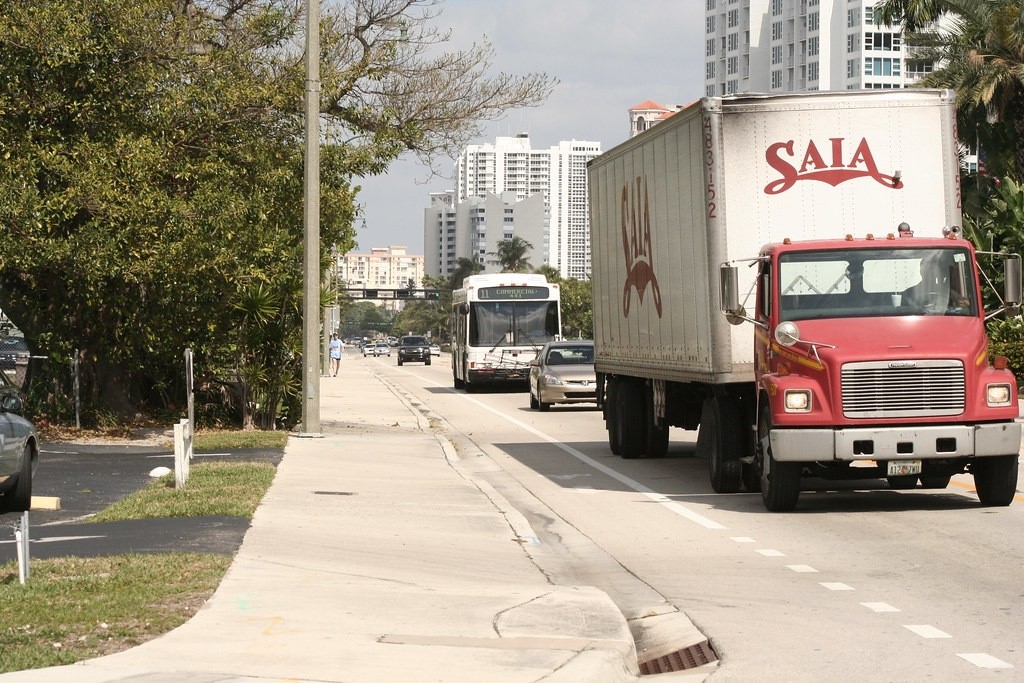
[0,392,43,523]
[430,343,441,358]
[526,339,605,413]
[397,336,432,365]
[351,336,399,350]
[374,343,392,357]
[364,344,378,355]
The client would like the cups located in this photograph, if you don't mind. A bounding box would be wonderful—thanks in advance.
[891,294,902,307]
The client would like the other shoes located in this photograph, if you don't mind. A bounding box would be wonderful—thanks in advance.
[333,374,336,378]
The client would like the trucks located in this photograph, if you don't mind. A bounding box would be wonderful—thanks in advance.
[585,86,1022,517]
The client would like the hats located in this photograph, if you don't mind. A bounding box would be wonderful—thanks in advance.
[333,332,338,335]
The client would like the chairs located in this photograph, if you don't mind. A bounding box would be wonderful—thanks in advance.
[547,351,564,364]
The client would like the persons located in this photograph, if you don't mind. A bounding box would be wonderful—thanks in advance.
[897,257,971,308]
[328,332,345,377]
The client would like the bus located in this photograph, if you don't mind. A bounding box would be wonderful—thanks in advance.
[448,271,567,392]
